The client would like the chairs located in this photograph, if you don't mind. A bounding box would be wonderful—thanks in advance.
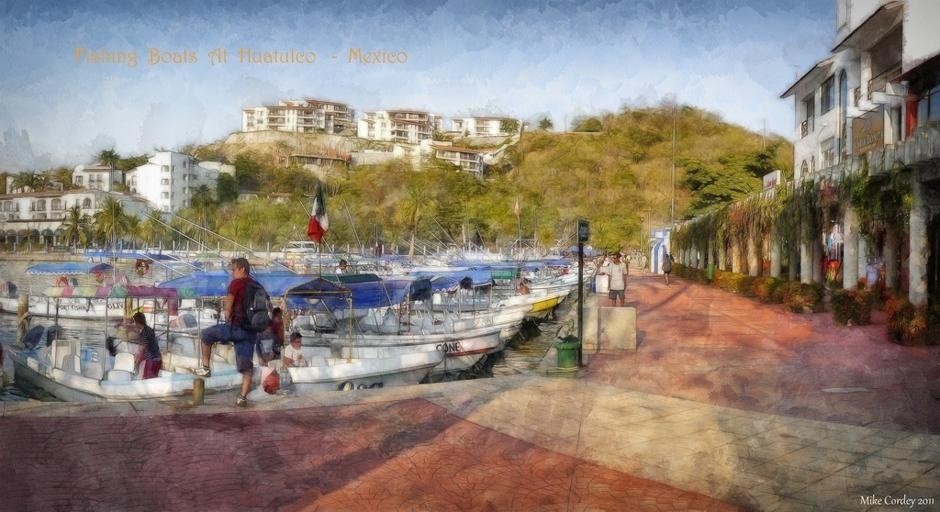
[60,348,136,385]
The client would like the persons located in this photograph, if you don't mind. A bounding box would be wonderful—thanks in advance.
[662,254,673,284]
[133,312,163,380]
[188,257,267,406]
[336,259,347,274]
[606,252,627,306]
[254,307,308,368]
[517,282,530,294]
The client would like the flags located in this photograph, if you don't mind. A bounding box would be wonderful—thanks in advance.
[308,181,330,249]
